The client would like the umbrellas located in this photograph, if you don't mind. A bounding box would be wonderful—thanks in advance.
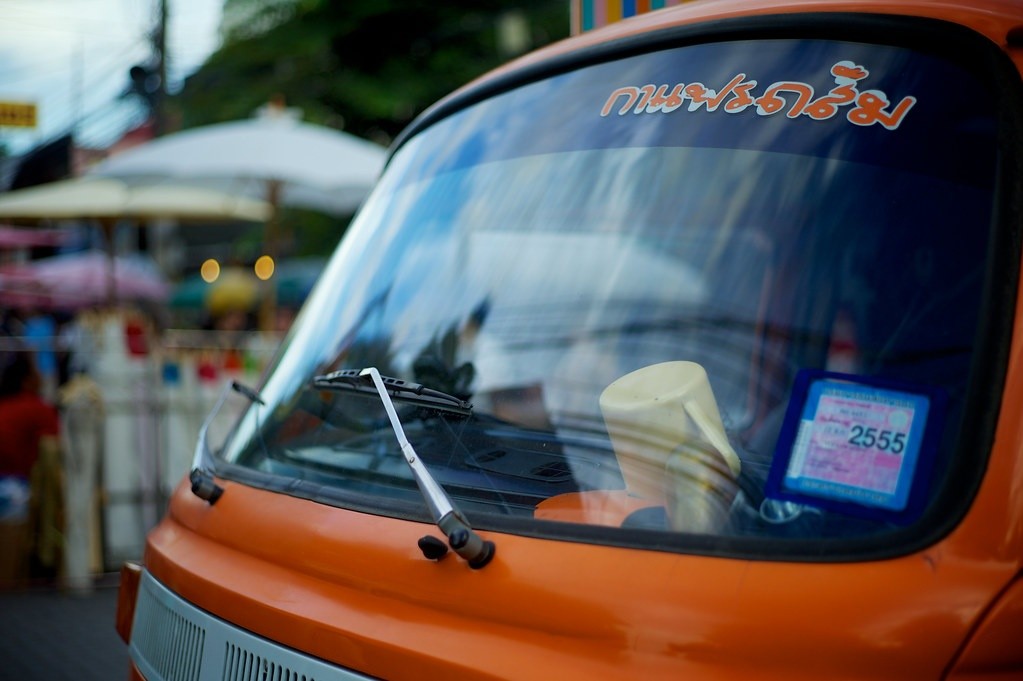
[0,101,393,332]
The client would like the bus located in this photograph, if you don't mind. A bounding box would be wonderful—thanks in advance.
[111,0,1023,681]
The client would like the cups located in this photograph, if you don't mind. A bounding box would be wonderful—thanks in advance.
[599,362,740,502]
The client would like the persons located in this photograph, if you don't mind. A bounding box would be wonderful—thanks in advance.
[0,357,69,592]
